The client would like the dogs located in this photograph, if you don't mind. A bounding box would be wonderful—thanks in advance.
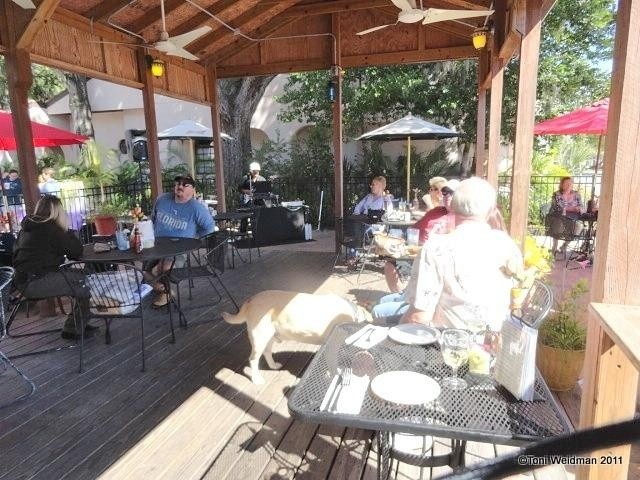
[221,290,374,385]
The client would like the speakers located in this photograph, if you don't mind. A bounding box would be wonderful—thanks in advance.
[124,129,148,162]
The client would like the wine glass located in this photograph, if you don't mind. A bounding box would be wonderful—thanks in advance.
[439,328,470,391]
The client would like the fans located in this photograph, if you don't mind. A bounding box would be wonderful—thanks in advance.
[352,0,496,37]
[86,0,213,61]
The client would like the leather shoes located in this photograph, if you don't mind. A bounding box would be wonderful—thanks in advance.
[84,323,101,331]
[61,331,93,340]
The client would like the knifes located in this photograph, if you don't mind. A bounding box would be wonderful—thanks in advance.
[321,366,345,413]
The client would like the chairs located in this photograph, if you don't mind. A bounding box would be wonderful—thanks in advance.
[0,197,599,480]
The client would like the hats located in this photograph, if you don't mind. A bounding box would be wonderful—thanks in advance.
[429,175,446,186]
[440,178,460,194]
[249,161,261,172]
[175,175,196,187]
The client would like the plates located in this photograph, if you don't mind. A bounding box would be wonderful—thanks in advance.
[370,370,443,406]
[388,322,440,346]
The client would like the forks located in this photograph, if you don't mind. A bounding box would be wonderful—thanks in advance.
[330,366,353,414]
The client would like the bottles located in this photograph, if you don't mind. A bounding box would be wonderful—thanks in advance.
[134,228,146,254]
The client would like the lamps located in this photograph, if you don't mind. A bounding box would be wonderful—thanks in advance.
[145,54,166,80]
[470,18,494,51]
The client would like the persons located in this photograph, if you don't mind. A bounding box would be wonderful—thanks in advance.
[12,194,102,339]
[238,161,267,237]
[37,166,59,200]
[138,171,216,308]
[0,169,24,204]
[418,176,447,215]
[544,176,585,258]
[352,176,396,242]
[370,207,507,324]
[384,178,464,290]
[395,174,523,330]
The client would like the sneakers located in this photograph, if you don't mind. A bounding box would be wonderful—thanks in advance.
[151,283,175,309]
[341,255,357,265]
[551,246,567,260]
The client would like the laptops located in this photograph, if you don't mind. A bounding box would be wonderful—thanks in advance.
[254,181,271,192]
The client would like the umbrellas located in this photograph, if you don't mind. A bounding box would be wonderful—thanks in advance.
[354,112,465,204]
[534,97,613,214]
[156,118,233,171]
[0,110,88,152]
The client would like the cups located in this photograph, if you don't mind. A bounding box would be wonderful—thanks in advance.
[115,231,130,251]
[389,228,403,239]
[137,220,155,249]
[406,228,420,248]
[398,201,405,212]
